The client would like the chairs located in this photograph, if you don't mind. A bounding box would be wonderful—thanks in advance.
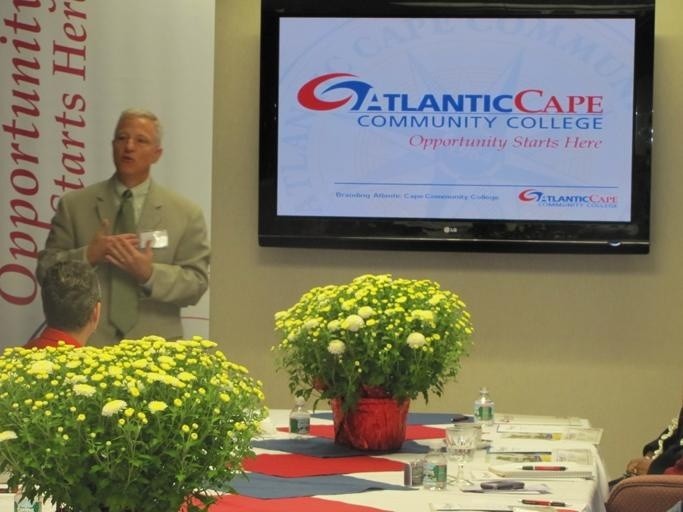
[604,474,683,512]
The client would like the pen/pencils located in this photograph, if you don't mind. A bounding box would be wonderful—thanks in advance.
[522,499,566,506]
[450,417,469,422]
[522,466,567,471]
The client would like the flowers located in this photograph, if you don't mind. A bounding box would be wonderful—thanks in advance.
[270,273,474,414]
[0,335,270,512]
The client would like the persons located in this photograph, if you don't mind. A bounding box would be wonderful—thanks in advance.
[42,106,211,348]
[23,261,100,348]
[665,497,682,512]
[607,405,682,489]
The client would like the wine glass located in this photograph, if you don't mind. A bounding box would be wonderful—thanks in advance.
[445,419,482,486]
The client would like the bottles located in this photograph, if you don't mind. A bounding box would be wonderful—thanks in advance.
[419,443,446,492]
[288,395,312,454]
[472,385,493,439]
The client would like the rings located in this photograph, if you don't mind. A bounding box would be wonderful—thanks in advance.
[119,257,125,263]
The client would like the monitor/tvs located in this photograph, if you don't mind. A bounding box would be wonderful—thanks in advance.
[257,0,656,253]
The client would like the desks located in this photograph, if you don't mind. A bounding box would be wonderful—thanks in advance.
[0,410,610,512]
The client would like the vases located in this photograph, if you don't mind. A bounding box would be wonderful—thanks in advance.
[330,388,410,451]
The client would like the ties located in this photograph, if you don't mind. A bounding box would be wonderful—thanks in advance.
[108,191,139,335]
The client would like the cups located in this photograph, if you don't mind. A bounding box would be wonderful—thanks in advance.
[403,460,426,490]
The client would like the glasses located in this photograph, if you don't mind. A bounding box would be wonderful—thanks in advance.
[446,474,473,487]
